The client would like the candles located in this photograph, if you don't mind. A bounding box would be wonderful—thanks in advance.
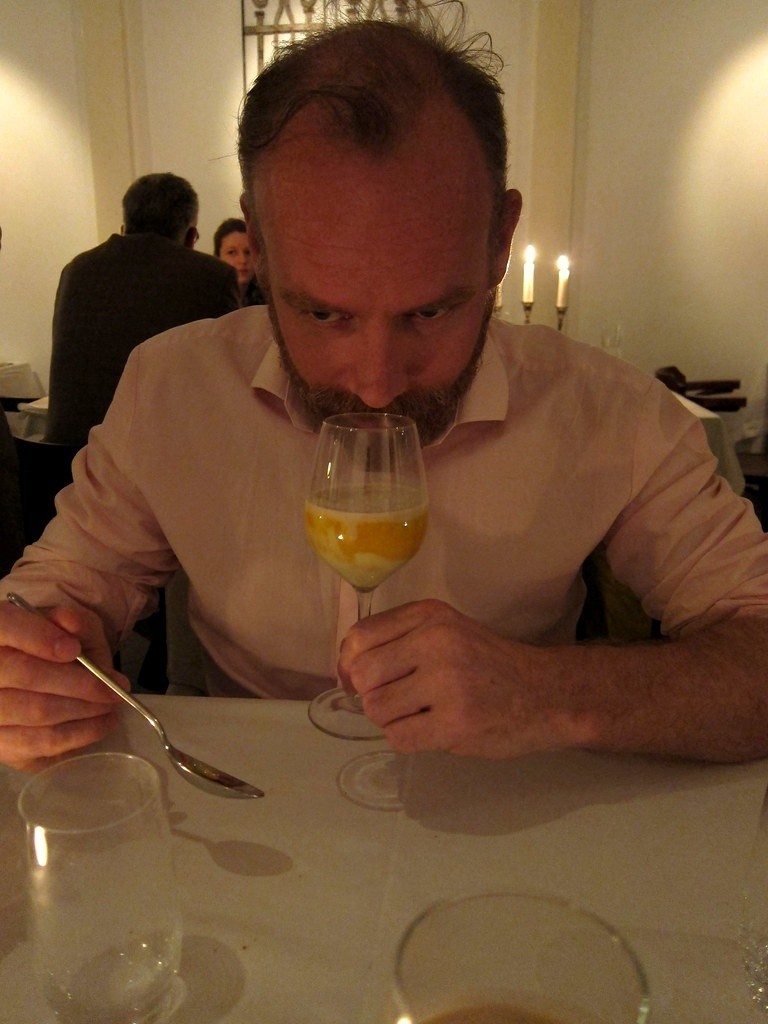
[522,245,536,302]
[495,284,503,307]
[556,255,570,309]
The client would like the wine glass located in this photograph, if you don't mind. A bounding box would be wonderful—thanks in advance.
[302,412,429,740]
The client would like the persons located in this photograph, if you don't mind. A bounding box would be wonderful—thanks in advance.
[42,173,241,701]
[213,215,268,310]
[1,19,767,778]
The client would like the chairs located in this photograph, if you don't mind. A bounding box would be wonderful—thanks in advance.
[655,367,768,537]
[12,434,171,695]
[1,362,44,413]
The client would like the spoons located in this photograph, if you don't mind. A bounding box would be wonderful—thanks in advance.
[5,592,265,800]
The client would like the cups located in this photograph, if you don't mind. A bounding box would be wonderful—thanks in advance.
[739,787,766,1007]
[17,752,184,1022]
[384,889,652,1023]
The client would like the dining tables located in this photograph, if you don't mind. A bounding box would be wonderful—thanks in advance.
[2,692,768,1023]
